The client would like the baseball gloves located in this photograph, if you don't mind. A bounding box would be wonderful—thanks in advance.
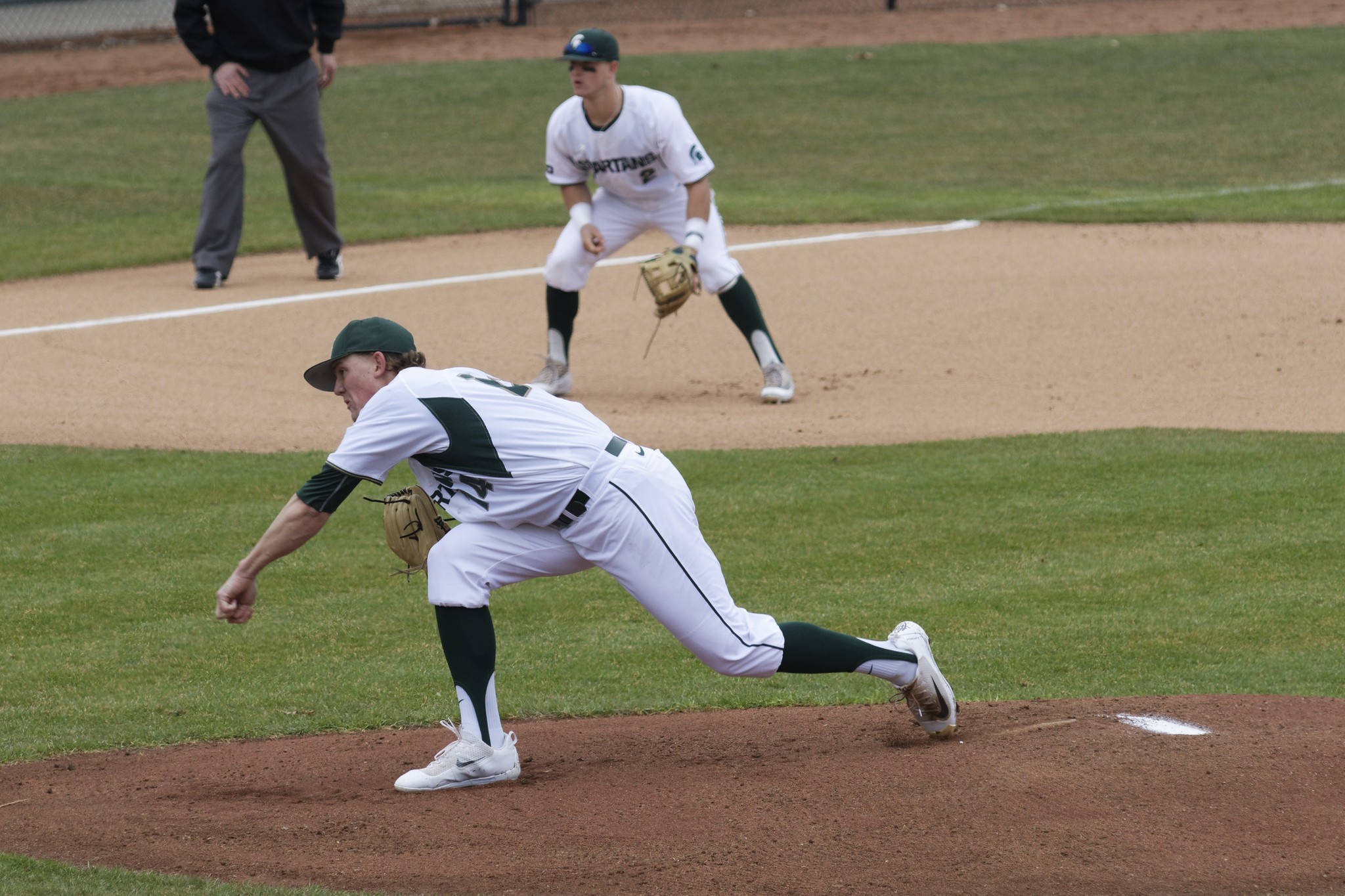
[637,243,703,320]
[381,483,454,574]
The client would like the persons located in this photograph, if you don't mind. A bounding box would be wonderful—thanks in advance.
[171,0,346,289]
[529,28,798,404]
[214,315,961,794]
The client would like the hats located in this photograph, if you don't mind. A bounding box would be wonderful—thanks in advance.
[303,317,419,392]
[556,28,618,64]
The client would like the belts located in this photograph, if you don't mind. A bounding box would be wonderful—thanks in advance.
[552,434,627,528]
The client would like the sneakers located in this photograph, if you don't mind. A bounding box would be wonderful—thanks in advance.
[315,248,341,279]
[193,267,221,287]
[393,717,521,792]
[886,620,957,738]
[761,362,793,402]
[524,350,571,394]
